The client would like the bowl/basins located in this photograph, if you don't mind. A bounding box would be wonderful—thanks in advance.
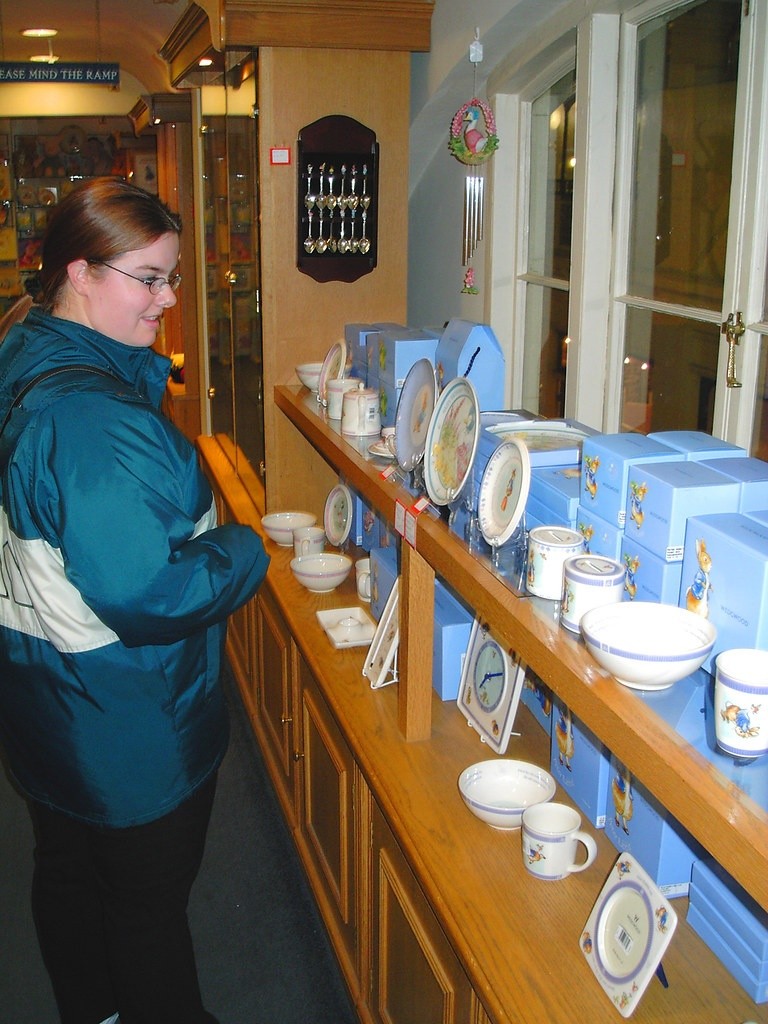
[295,362,352,393]
[289,553,352,593]
[458,758,556,831]
[580,601,717,691]
[261,512,315,546]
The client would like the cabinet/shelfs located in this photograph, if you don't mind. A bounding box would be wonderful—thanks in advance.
[196,435,768,1024]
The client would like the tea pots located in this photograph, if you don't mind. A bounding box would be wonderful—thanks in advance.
[341,383,381,436]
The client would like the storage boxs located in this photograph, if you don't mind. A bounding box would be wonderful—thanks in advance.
[340,318,768,1005]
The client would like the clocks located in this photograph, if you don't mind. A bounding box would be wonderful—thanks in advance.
[473,640,508,713]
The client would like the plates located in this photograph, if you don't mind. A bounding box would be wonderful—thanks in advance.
[319,339,346,402]
[324,484,352,547]
[424,378,480,506]
[367,440,396,459]
[395,359,439,472]
[374,442,390,454]
[478,437,531,547]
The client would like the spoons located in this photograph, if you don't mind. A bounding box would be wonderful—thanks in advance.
[304,210,370,254]
[304,163,371,210]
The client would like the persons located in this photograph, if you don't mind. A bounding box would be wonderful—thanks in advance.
[0,177,272,1023]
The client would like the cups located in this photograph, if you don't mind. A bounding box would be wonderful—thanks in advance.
[521,803,598,881]
[326,380,361,419]
[382,428,395,447]
[713,649,768,758]
[526,527,584,601]
[560,554,626,635]
[293,528,324,557]
[355,557,371,602]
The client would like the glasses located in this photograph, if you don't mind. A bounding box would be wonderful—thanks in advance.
[100,259,182,296]
